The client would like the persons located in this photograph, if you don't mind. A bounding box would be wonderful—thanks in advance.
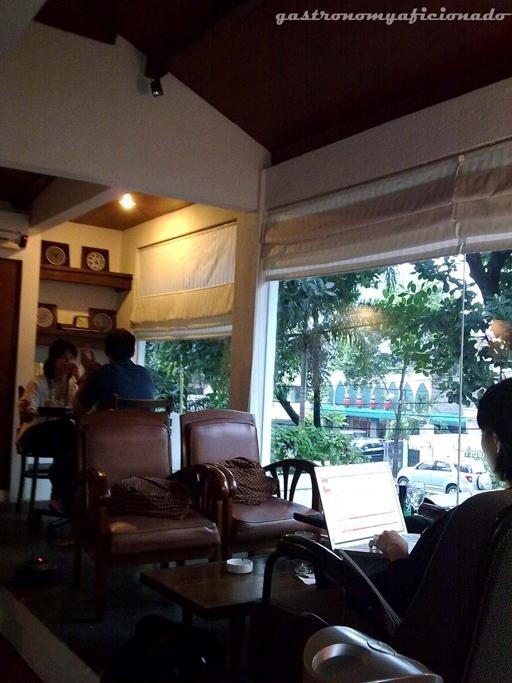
[367,377,511,681]
[72,329,156,415]
[18,339,89,512]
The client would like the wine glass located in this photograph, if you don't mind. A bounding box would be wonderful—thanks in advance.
[295,532,314,574]
[405,481,425,515]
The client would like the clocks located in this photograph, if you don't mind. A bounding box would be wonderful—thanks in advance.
[89,307,116,334]
[37,302,57,328]
[81,245,109,270]
[41,240,70,265]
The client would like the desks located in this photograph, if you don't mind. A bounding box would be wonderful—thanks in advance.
[139,546,420,660]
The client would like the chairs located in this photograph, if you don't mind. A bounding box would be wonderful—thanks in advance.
[77,408,223,615]
[19,383,27,398]
[180,409,328,557]
[15,416,75,517]
[260,520,512,683]
[96,393,176,415]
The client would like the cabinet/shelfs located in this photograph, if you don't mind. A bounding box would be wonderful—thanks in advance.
[37,268,133,345]
[0,258,23,492]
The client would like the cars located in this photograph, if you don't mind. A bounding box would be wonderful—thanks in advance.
[348,438,387,462]
[399,461,492,498]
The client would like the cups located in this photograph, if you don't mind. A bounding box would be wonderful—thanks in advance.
[45,386,58,407]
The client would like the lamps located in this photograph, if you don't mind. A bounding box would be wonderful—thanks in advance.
[118,193,136,210]
[144,57,169,96]
